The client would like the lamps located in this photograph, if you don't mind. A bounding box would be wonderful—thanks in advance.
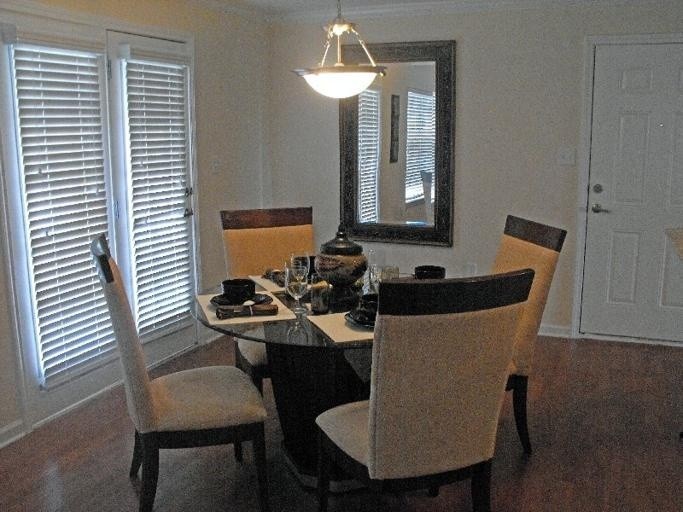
[291,0,388,99]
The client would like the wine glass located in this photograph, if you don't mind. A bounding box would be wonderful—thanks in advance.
[286,306,309,347]
[289,251,310,285]
[284,265,309,311]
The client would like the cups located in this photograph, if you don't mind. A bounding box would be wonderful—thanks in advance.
[310,273,329,314]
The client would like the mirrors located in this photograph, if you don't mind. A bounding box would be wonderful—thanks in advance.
[338,40,457,248]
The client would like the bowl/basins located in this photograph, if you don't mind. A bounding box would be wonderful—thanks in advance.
[361,293,378,314]
[414,265,445,279]
[220,278,255,303]
[293,256,317,275]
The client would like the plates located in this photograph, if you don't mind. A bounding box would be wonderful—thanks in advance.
[345,313,376,329]
[208,292,273,308]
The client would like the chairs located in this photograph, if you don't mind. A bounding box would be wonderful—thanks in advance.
[220,206,327,464]
[91,232,268,512]
[314,268,535,512]
[420,171,434,221]
[492,214,568,454]
